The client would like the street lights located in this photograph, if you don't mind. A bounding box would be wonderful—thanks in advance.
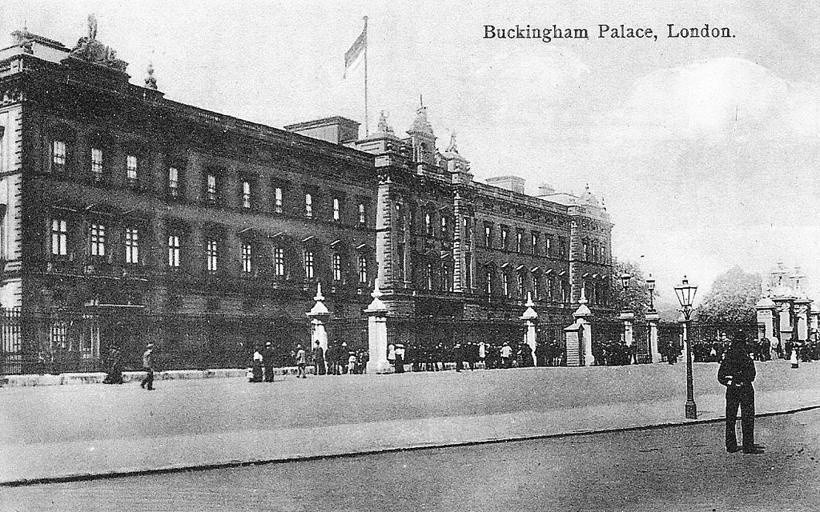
[644,273,662,364]
[673,274,698,420]
[618,270,635,364]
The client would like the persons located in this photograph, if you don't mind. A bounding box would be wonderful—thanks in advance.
[691,338,820,362]
[716,334,767,456]
[665,340,674,364]
[590,338,640,366]
[140,343,157,391]
